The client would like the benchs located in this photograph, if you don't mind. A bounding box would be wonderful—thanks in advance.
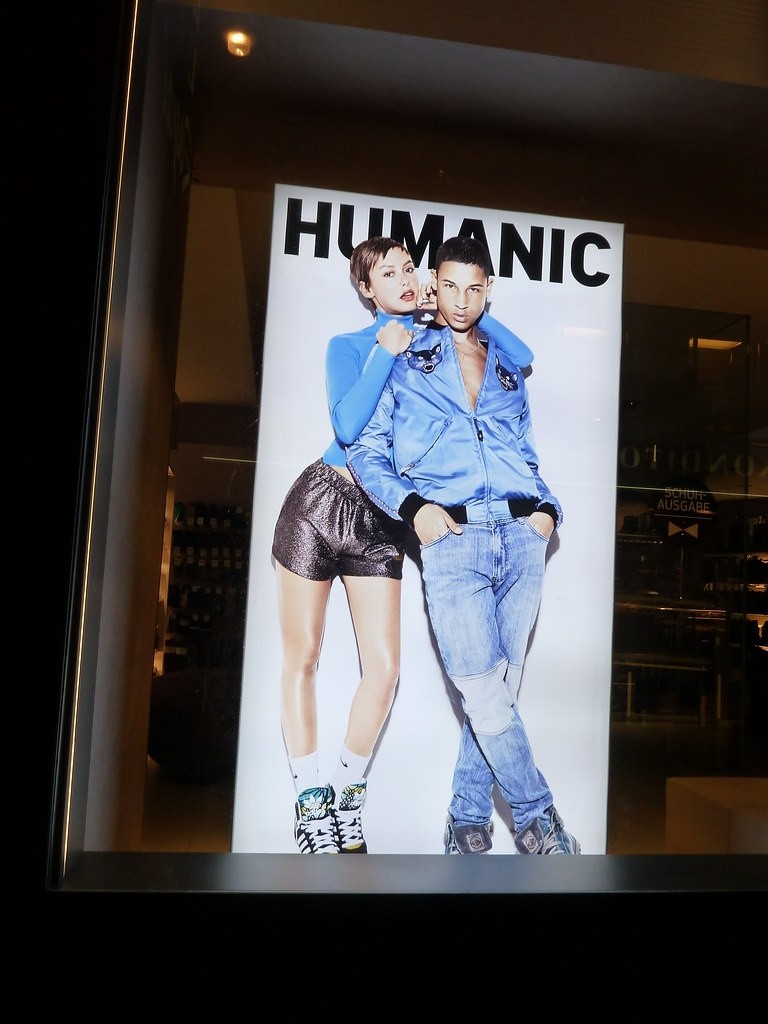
[615,649,716,730]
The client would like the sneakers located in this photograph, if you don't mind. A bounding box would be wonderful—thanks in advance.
[328,778,367,853]
[294,786,340,855]
[443,814,494,855]
[514,804,581,857]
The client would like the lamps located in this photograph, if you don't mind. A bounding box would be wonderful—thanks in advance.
[686,334,744,352]
[226,29,256,60]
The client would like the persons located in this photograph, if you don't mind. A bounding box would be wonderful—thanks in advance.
[345,239,582,855]
[272,237,440,854]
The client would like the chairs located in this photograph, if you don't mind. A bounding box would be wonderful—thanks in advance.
[149,667,242,787]
